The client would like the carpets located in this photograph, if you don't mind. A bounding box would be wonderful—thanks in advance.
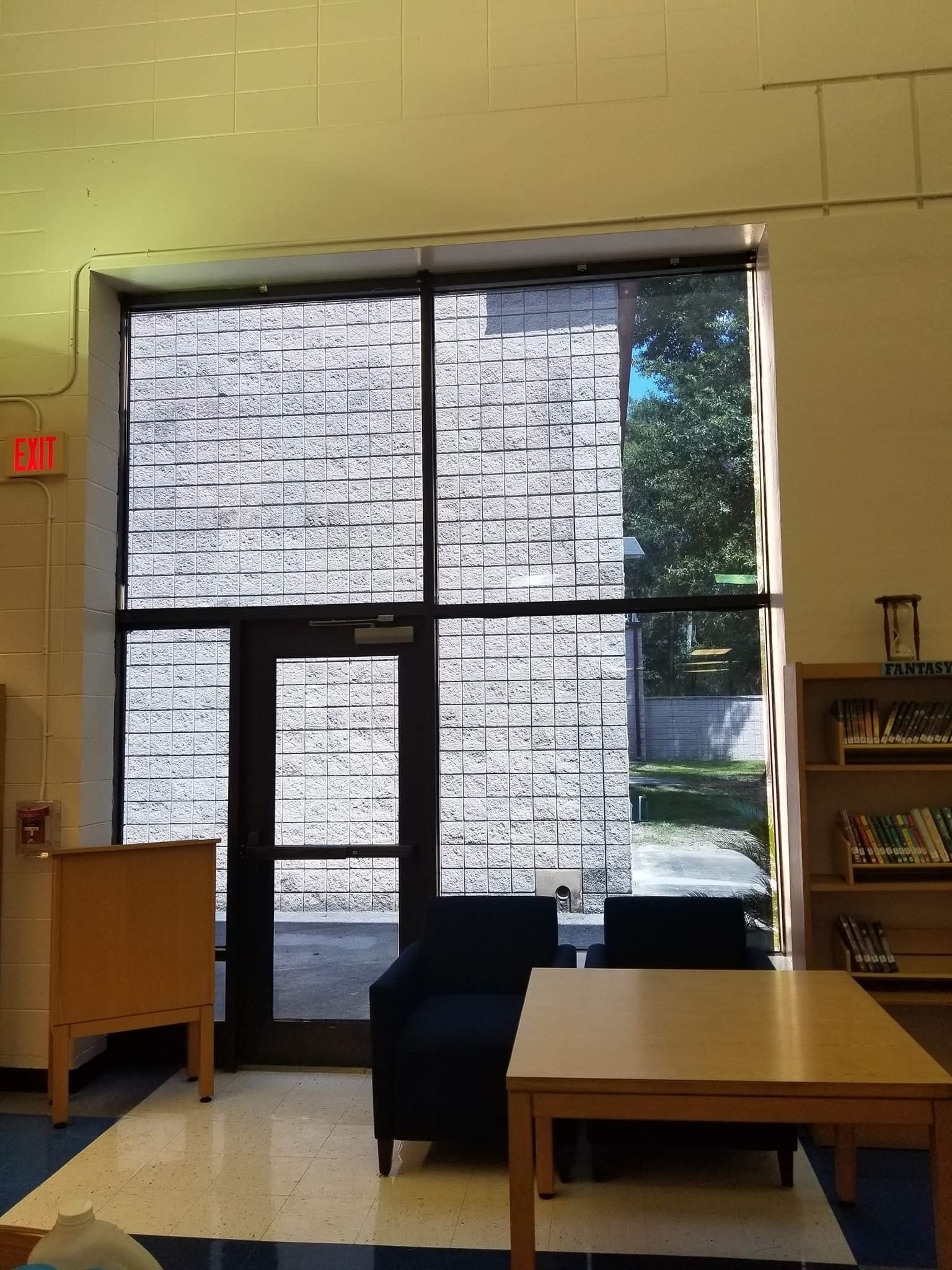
[799,1122,936,1270]
[0,1113,120,1218]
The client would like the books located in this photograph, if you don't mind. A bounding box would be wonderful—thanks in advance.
[829,697,952,745]
[837,912,899,973]
[836,808,952,864]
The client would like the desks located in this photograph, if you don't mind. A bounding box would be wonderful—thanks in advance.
[507,966,951,1268]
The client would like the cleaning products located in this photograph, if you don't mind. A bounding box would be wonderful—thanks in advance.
[25,1198,163,1270]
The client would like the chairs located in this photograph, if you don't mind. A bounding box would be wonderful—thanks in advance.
[370,896,578,1185]
[574,898,800,1190]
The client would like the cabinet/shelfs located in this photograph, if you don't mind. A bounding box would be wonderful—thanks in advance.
[783,659,952,1003]
